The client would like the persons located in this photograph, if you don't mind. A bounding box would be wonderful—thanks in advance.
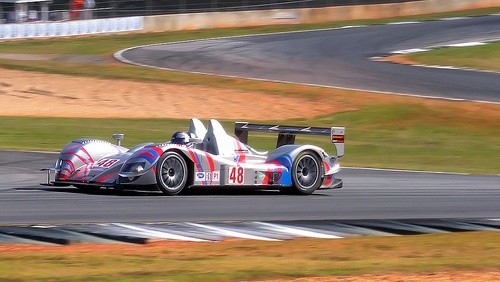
[70,0,95,21]
[171,132,193,148]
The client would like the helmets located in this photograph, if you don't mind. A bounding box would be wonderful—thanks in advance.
[171,131,190,145]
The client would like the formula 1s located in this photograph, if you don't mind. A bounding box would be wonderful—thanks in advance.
[40,117,345,195]
